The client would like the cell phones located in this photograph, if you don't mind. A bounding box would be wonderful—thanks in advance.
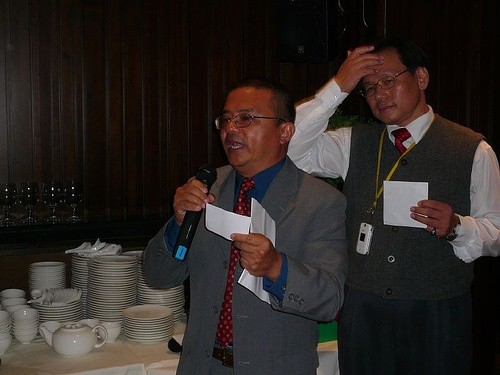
[355,222,374,256]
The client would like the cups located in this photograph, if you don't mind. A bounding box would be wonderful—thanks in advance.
[39,321,60,338]
[98,322,121,343]
[79,319,98,342]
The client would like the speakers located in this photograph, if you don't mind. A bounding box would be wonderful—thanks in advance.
[276,0,373,63]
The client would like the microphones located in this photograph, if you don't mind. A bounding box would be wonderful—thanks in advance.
[171,164,217,262]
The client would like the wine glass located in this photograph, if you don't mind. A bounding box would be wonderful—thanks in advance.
[42,176,65,223]
[0,176,17,224]
[63,176,85,222]
[17,177,41,223]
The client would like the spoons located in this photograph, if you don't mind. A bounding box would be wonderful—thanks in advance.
[26,287,82,305]
[65,238,122,257]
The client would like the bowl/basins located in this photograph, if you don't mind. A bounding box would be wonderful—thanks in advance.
[0,289,40,356]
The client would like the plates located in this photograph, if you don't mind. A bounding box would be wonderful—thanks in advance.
[28,250,185,345]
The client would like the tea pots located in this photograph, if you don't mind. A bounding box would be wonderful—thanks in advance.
[39,322,108,358]
